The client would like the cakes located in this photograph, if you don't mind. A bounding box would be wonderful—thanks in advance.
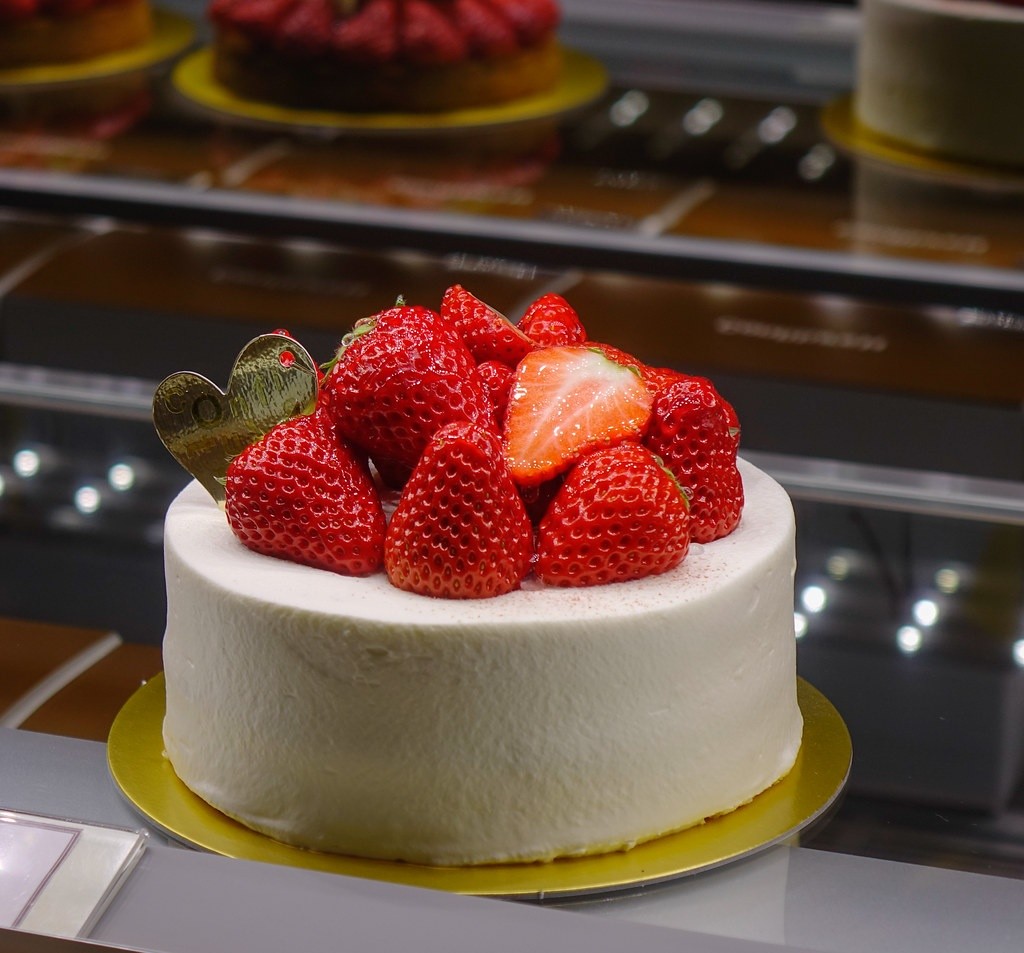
[153,285,804,866]
[0,1,156,68]
[858,0,1024,168]
[210,0,562,113]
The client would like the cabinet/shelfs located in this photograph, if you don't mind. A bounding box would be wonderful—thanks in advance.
[0,6,1023,953]
